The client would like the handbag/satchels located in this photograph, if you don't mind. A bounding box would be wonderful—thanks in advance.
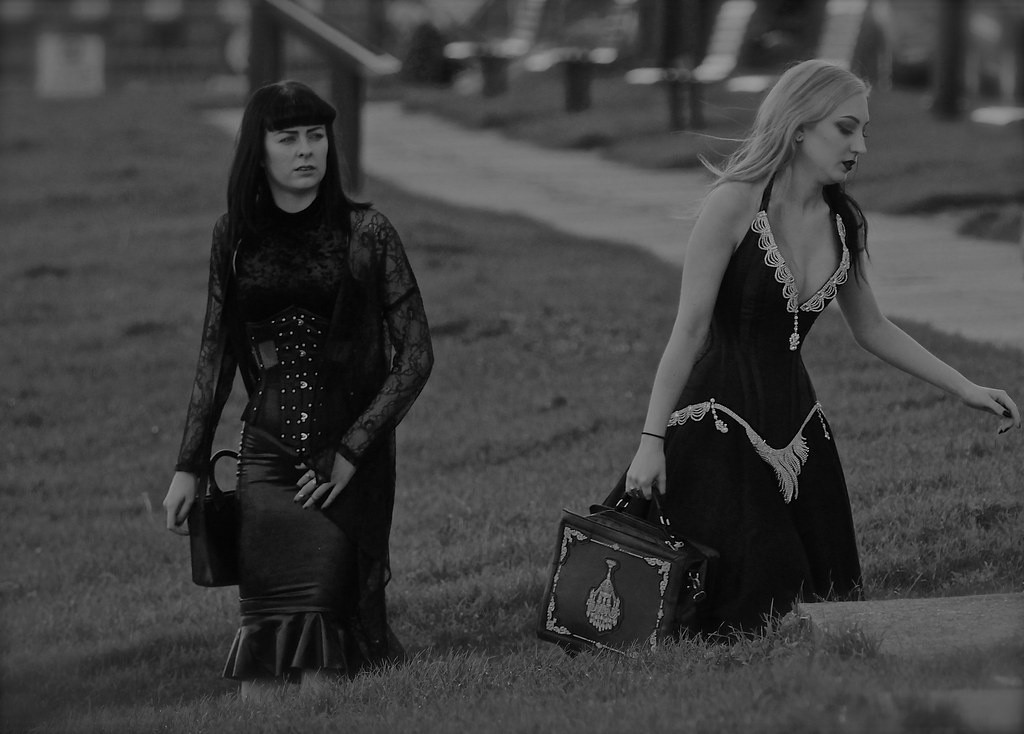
[185,449,240,588]
[536,486,720,665]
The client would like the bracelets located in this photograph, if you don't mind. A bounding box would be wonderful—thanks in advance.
[641,432,666,441]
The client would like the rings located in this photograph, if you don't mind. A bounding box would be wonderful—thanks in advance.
[305,473,311,479]
[310,480,318,487]
[630,488,642,496]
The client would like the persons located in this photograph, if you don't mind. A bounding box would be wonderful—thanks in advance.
[162,80,435,702]
[604,60,1022,644]
